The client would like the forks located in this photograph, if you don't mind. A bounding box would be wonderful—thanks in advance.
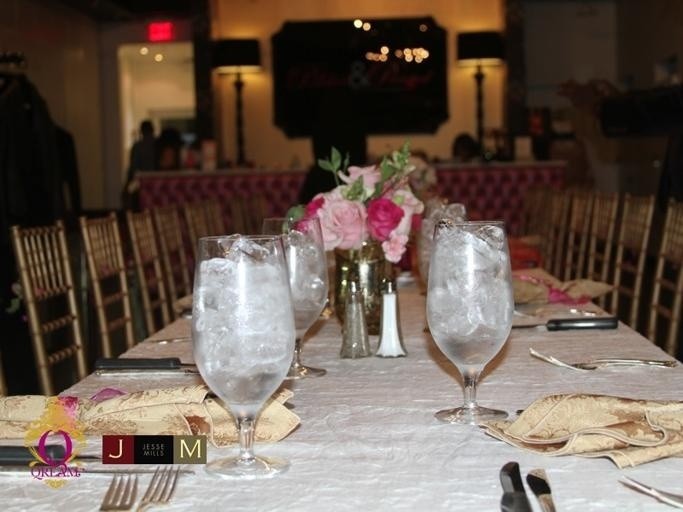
[526,349,677,374]
[97,474,138,511]
[135,463,183,512]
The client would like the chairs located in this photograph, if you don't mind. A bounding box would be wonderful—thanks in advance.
[9,192,274,396]
[508,184,682,360]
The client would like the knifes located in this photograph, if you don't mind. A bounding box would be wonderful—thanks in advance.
[499,459,530,511]
[525,467,555,511]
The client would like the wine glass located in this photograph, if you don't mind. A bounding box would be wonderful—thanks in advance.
[190,233,298,487]
[423,218,516,425]
[260,215,333,379]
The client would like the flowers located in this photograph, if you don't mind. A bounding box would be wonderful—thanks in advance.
[280,145,412,260]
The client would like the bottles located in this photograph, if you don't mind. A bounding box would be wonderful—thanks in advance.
[373,278,409,358]
[338,279,373,358]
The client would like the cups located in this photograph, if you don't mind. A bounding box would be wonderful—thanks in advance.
[414,211,465,281]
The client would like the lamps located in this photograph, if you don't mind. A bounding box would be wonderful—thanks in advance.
[455,28,506,167]
[214,35,264,171]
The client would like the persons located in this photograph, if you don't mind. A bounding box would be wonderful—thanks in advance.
[451,132,485,162]
[124,121,191,213]
[0,50,82,397]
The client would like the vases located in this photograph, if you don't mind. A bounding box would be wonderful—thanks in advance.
[331,241,388,337]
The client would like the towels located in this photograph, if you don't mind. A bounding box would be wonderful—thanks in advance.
[488,392,682,468]
[508,270,612,306]
[1,371,300,450]
